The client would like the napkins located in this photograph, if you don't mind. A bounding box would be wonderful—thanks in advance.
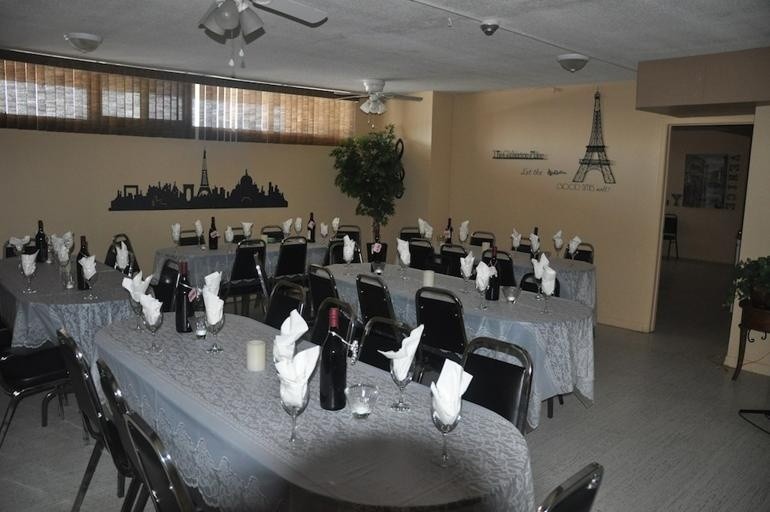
[1,216,598,428]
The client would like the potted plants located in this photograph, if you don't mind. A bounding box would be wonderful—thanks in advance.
[729,255,770,309]
[328,122,403,262]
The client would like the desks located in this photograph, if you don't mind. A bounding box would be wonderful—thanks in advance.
[732,299,770,380]
[93,310,537,511]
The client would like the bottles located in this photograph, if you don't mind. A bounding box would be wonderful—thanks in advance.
[306,212,316,243]
[530,227,541,262]
[370,234,385,275]
[443,217,453,247]
[175,261,195,333]
[76,235,91,291]
[320,307,347,411]
[209,216,218,249]
[485,247,501,301]
[36,219,49,262]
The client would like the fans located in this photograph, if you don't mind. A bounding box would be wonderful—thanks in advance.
[220,0,329,24]
[333,79,423,102]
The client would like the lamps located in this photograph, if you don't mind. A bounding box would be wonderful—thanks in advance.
[63,32,102,54]
[557,54,589,73]
[198,0,264,36]
[360,94,387,128]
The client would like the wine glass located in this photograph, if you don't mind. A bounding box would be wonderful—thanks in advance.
[278,358,464,468]
[170,224,302,250]
[14,246,99,300]
[342,253,555,316]
[459,231,579,264]
[129,294,225,355]
[320,233,328,245]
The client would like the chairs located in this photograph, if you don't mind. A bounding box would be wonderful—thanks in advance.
[536,460,603,512]
[56,327,127,511]
[664,213,678,259]
[123,409,197,511]
